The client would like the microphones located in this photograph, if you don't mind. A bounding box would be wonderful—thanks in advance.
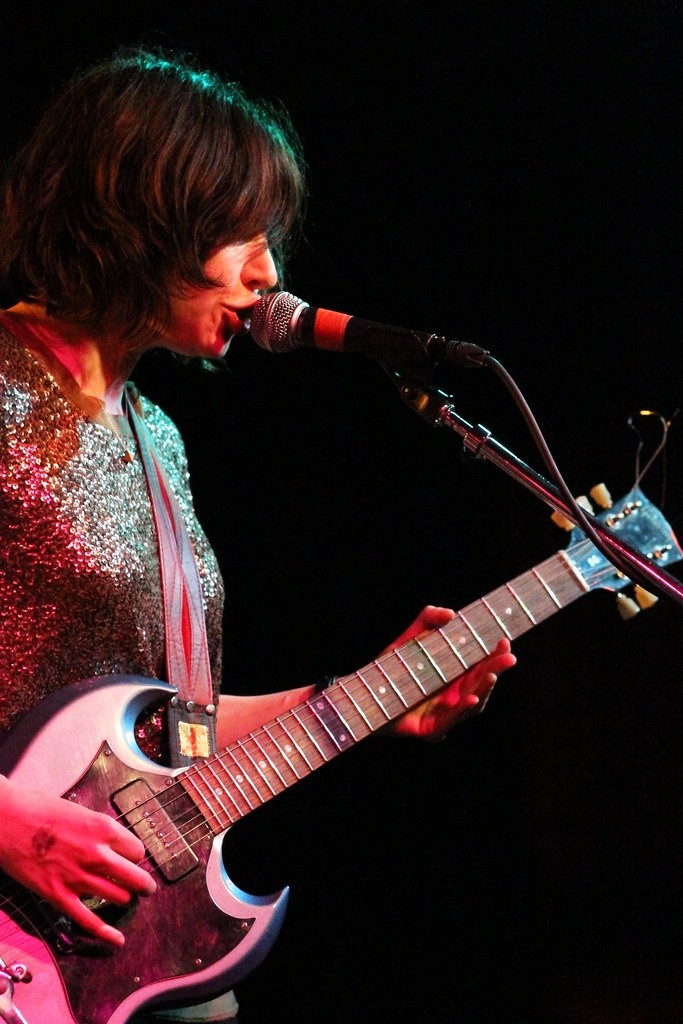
[249,290,491,367]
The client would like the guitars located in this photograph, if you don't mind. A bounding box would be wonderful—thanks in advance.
[0,411,683,1023]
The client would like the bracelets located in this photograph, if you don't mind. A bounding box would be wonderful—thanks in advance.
[314,675,335,695]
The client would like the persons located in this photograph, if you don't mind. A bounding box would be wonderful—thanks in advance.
[0,45,518,1024]
